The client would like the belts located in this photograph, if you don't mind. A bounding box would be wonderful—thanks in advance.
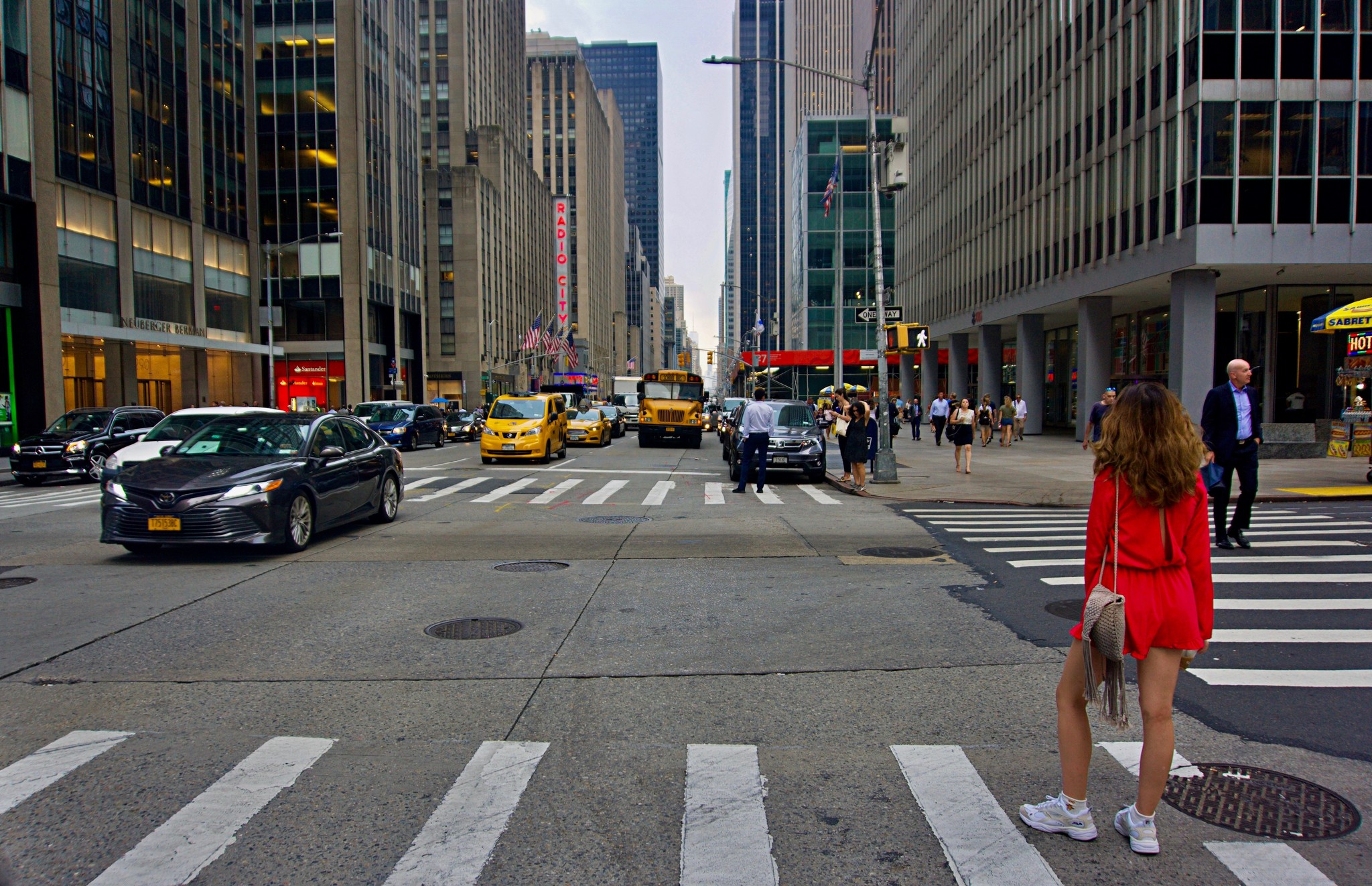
[1235,438,1253,445]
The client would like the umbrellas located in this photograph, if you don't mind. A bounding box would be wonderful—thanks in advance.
[1310,297,1372,334]
[820,385,834,395]
[430,397,450,409]
[842,382,854,390]
[846,384,870,402]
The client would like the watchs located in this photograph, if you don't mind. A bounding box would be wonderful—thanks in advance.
[1025,416,1027,418]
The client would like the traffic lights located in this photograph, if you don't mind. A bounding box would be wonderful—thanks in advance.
[677,354,684,367]
[884,324,909,351]
[907,325,930,349]
[706,351,713,365]
[739,357,745,370]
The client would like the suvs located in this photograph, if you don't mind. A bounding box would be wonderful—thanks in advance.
[9,405,169,486]
[727,399,830,481]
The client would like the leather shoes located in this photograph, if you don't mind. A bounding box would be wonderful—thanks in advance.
[1216,526,1251,549]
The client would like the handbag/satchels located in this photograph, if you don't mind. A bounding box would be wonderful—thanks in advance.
[1081,583,1131,733]
[831,423,836,435]
[893,421,902,435]
[897,405,904,420]
[836,402,850,437]
[945,408,962,442]
[903,408,908,413]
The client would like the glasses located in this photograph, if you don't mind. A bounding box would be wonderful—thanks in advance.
[1104,387,1116,392]
[852,409,857,412]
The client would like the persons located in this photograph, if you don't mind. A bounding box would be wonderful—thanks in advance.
[1200,359,1262,548]
[440,401,493,421]
[1018,383,1217,852]
[732,389,774,493]
[805,388,1028,491]
[131,401,138,406]
[1083,388,1116,450]
[190,399,354,416]
[3,396,12,421]
[1286,388,1305,423]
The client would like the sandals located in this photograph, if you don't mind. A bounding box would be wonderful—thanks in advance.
[965,466,971,474]
[956,465,961,472]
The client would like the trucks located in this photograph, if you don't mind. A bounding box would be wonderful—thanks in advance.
[607,376,642,431]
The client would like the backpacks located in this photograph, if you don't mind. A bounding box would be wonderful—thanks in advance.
[347,408,352,415]
[979,409,990,425]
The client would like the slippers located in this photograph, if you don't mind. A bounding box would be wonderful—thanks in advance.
[835,476,852,482]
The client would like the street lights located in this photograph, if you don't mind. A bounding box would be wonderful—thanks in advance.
[719,283,772,402]
[701,53,902,483]
[562,349,589,382]
[266,232,345,408]
[532,335,567,391]
[587,357,610,400]
[488,320,500,392]
[600,363,622,402]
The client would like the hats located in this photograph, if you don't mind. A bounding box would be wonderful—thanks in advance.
[440,408,444,411]
[287,405,291,407]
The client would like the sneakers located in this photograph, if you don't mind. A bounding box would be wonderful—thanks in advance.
[1114,804,1160,854]
[1020,792,1098,841]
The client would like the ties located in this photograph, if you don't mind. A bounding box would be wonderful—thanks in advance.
[914,405,917,417]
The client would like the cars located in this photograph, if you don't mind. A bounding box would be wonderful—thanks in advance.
[701,397,753,463]
[354,401,485,451]
[97,406,300,497]
[100,412,403,558]
[479,392,628,465]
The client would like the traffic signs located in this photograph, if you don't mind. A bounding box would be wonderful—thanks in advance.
[531,375,540,378]
[854,305,904,324]
[484,379,497,384]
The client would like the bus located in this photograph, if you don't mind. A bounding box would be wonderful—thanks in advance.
[636,371,710,449]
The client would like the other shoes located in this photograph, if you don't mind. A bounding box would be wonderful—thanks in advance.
[732,488,746,493]
[849,483,866,491]
[911,429,941,446]
[980,435,1023,447]
[902,422,906,424]
[756,489,763,493]
